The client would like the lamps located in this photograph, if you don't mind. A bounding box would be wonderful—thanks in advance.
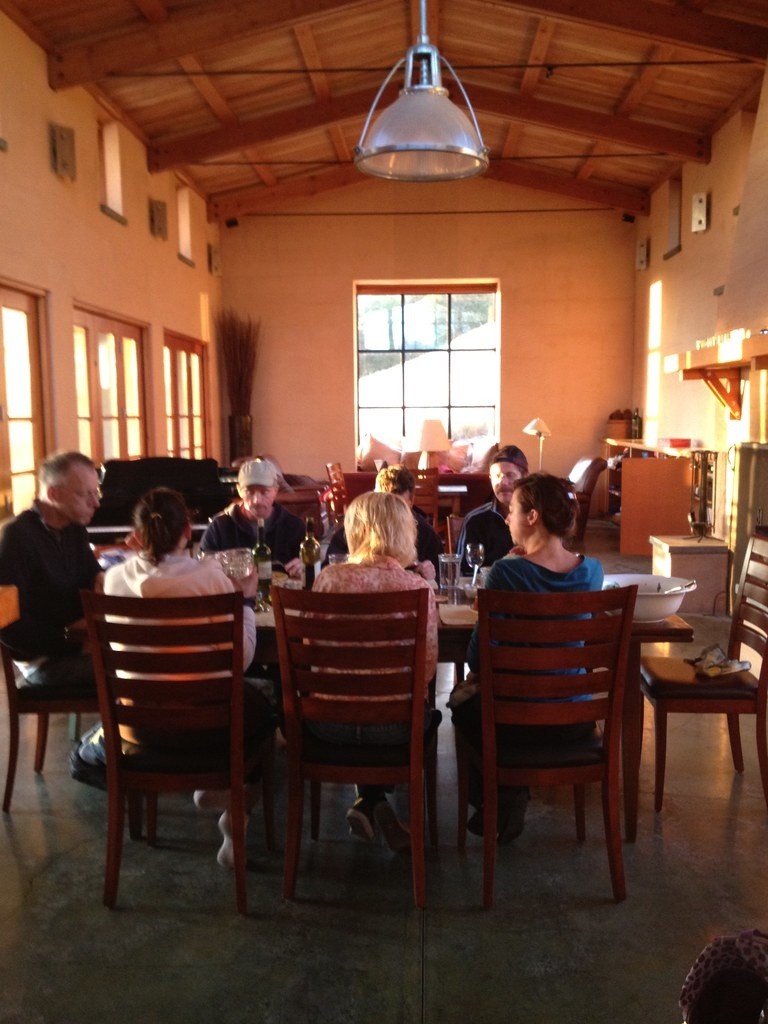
[352,0,490,182]
[418,418,450,468]
[522,417,551,469]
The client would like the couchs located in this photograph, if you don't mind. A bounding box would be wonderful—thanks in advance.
[562,453,608,548]
[232,454,329,539]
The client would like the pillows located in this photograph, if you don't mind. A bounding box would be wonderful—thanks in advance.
[358,435,475,474]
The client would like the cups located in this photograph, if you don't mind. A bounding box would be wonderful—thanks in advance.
[328,554,348,565]
[480,567,491,587]
[438,554,462,589]
[441,589,461,606]
[225,547,252,578]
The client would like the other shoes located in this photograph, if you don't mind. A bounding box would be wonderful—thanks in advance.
[346,793,412,887]
[465,774,528,844]
[70,741,154,795]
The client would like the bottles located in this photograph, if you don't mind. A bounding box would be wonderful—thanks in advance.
[251,518,273,609]
[632,408,641,439]
[299,517,321,592]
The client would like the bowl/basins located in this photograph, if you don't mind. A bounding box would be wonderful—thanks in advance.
[601,573,697,623]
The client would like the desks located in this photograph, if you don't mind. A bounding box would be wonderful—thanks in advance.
[86,524,210,559]
[126,595,693,841]
[438,485,470,515]
[346,472,490,507]
[651,536,729,617]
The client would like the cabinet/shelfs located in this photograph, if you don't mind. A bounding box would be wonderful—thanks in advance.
[600,437,712,558]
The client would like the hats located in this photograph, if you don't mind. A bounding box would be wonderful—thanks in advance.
[237,461,278,487]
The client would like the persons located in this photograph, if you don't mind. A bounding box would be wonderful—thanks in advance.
[445,469,605,848]
[322,464,445,584]
[456,444,528,578]
[72,486,279,873]
[197,458,304,579]
[300,492,443,841]
[0,452,121,793]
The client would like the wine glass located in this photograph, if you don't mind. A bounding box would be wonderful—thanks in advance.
[466,543,484,583]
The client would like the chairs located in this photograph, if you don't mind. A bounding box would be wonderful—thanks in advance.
[326,461,347,531]
[228,414,253,464]
[0,645,102,814]
[77,584,638,916]
[639,533,768,814]
[410,467,438,533]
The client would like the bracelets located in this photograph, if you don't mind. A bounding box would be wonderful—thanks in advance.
[244,598,257,609]
